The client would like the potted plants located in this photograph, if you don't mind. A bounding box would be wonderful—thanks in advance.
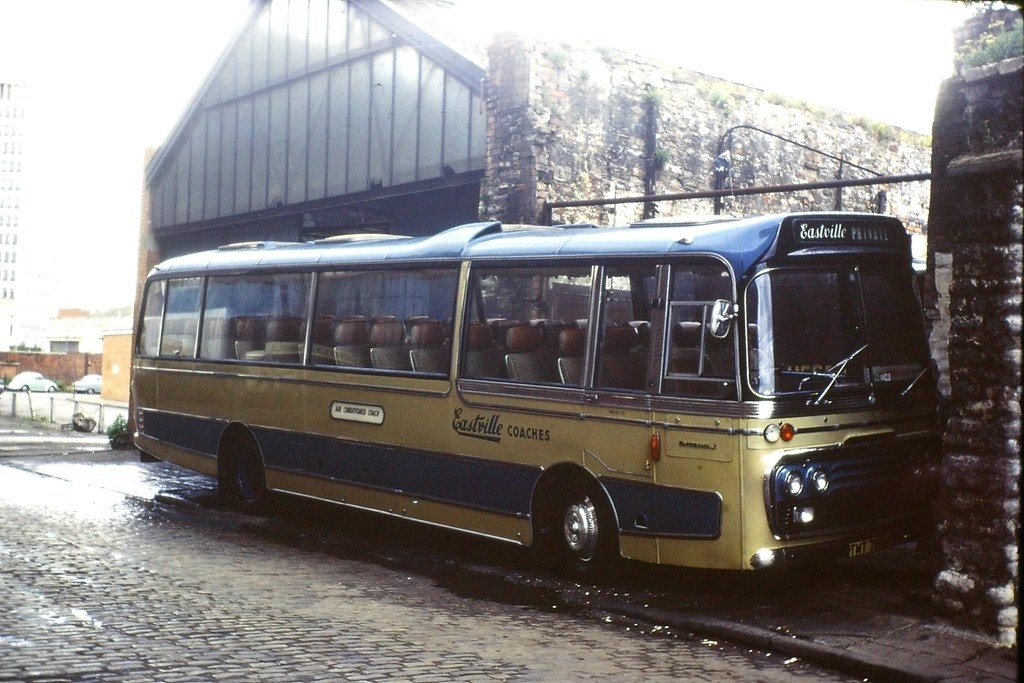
[107,414,129,450]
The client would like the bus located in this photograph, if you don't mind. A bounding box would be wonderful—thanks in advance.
[127,211,950,588]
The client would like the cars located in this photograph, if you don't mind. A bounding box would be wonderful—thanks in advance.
[7,371,59,393]
[71,374,102,394]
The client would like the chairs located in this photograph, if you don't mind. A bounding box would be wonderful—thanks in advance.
[601,326,641,390]
[669,321,722,399]
[556,328,606,388]
[626,320,651,330]
[574,319,588,327]
[493,319,519,344]
[234,318,266,361]
[369,321,407,371]
[266,315,301,362]
[140,315,228,357]
[504,326,552,384]
[297,315,335,364]
[333,319,369,367]
[409,321,446,372]
[465,323,504,377]
